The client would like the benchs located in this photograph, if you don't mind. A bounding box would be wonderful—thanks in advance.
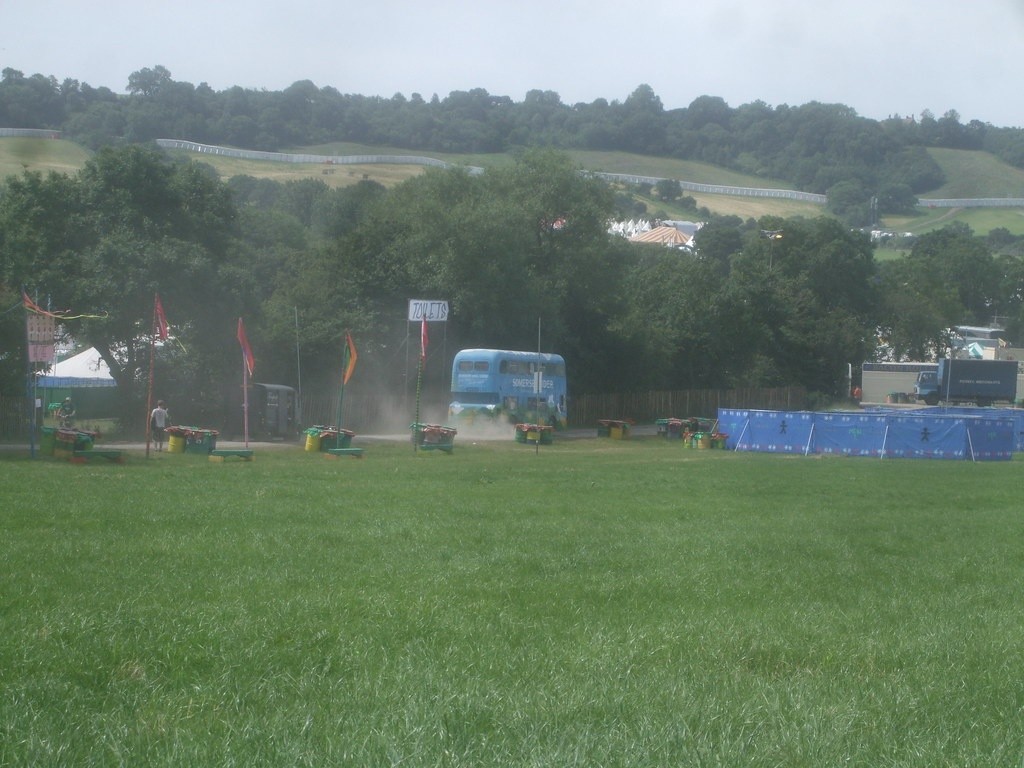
[209,450,256,463]
[71,451,125,464]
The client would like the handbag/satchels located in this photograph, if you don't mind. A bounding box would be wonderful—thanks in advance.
[151,416,155,429]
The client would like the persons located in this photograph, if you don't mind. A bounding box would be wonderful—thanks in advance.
[57,396,75,427]
[151,400,168,452]
[854,386,861,405]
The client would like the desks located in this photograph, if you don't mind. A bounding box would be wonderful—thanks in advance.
[416,444,454,456]
[324,448,364,460]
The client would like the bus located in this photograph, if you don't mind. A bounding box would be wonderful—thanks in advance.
[448,349,569,433]
[246,383,303,442]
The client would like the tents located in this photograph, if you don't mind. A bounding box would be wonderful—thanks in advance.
[31,325,177,426]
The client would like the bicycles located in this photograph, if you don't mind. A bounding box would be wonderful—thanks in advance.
[56,413,74,430]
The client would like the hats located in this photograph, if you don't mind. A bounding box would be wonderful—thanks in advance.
[66,397,71,401]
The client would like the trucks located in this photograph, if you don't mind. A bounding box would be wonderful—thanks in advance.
[915,358,1018,406]
